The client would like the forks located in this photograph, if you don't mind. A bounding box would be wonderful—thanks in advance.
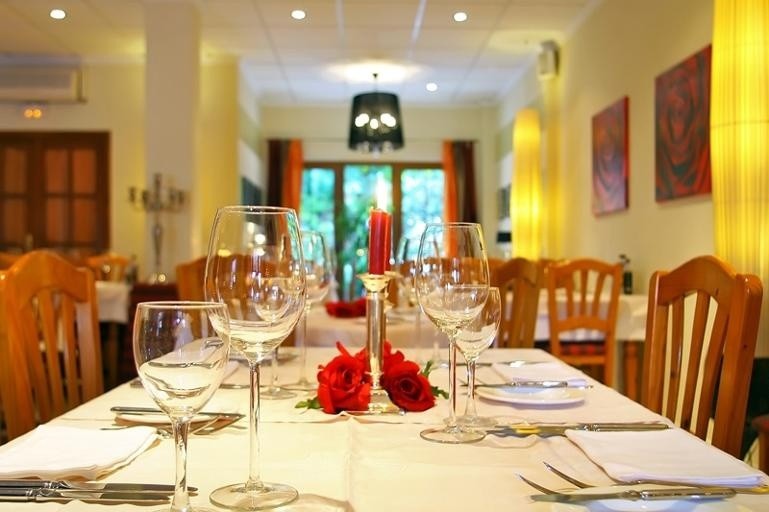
[518,460,765,504]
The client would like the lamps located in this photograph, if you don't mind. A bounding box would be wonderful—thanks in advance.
[348,73,405,154]
[536,43,556,79]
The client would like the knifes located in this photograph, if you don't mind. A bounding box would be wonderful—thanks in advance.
[114,409,242,417]
[443,358,560,368]
[0,478,204,506]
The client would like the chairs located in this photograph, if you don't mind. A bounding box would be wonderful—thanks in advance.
[176,255,251,342]
[546,260,624,386]
[1,251,106,444]
[388,256,576,349]
[641,255,761,458]
[87,253,127,387]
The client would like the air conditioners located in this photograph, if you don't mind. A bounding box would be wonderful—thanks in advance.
[1,52,88,105]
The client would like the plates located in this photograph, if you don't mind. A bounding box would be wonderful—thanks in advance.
[121,414,218,425]
[477,380,591,411]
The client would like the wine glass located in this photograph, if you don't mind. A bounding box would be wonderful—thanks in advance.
[259,230,330,398]
[441,285,500,431]
[203,205,307,510]
[414,223,490,444]
[133,302,229,512]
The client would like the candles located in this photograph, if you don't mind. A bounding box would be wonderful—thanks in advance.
[370,184,391,274]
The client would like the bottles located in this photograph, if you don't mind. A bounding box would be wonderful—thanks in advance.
[625,259,634,296]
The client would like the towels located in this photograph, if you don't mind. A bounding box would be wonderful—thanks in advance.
[0,425,163,480]
[565,429,764,488]
